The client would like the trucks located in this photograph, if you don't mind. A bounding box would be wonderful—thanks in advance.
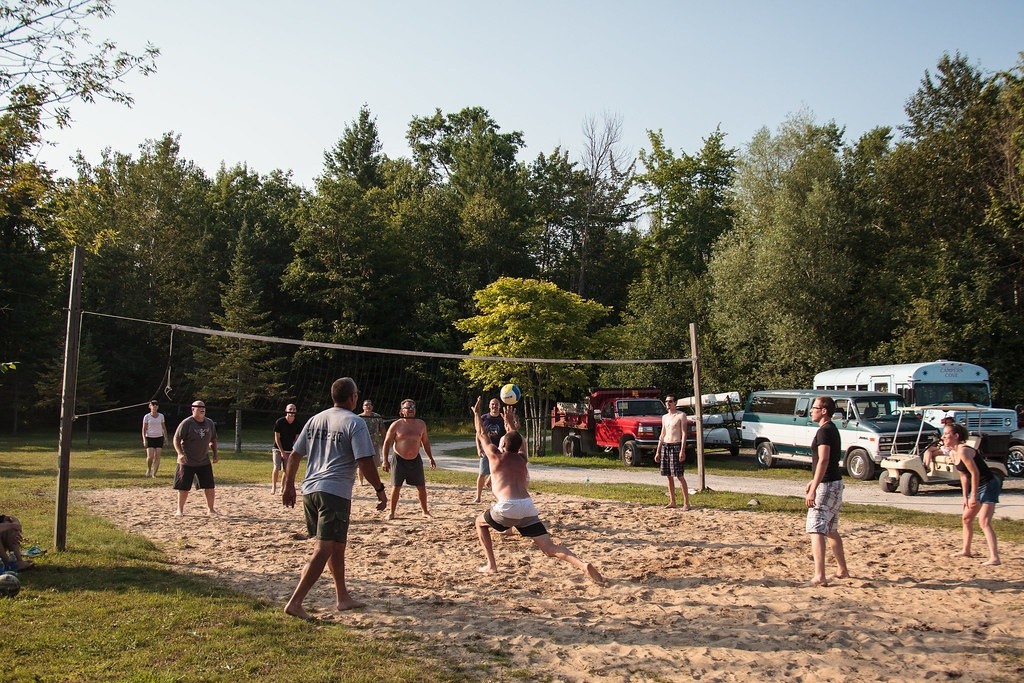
[551,386,697,467]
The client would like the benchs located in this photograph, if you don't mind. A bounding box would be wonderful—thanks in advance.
[933,436,980,463]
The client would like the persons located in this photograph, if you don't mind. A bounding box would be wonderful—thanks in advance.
[803,397,849,586]
[282,377,388,621]
[471,395,603,583]
[141,399,167,479]
[922,418,1001,566]
[654,394,691,511]
[0,515,34,570]
[271,404,302,495]
[384,399,437,520]
[173,400,219,517]
[357,399,386,486]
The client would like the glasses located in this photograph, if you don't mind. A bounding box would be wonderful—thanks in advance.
[402,405,416,409]
[811,405,822,410]
[664,400,674,403]
[351,390,361,395]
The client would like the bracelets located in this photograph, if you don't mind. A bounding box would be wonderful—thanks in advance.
[375,483,385,492]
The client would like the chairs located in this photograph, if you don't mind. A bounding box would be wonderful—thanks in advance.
[862,406,878,418]
[835,407,846,419]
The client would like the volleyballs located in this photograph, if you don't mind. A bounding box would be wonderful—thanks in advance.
[0,574,20,596]
[500,383,521,405]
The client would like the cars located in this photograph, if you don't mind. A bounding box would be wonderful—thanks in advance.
[1005,428,1024,477]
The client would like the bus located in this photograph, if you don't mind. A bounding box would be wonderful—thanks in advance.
[741,389,942,481]
[814,360,1018,457]
